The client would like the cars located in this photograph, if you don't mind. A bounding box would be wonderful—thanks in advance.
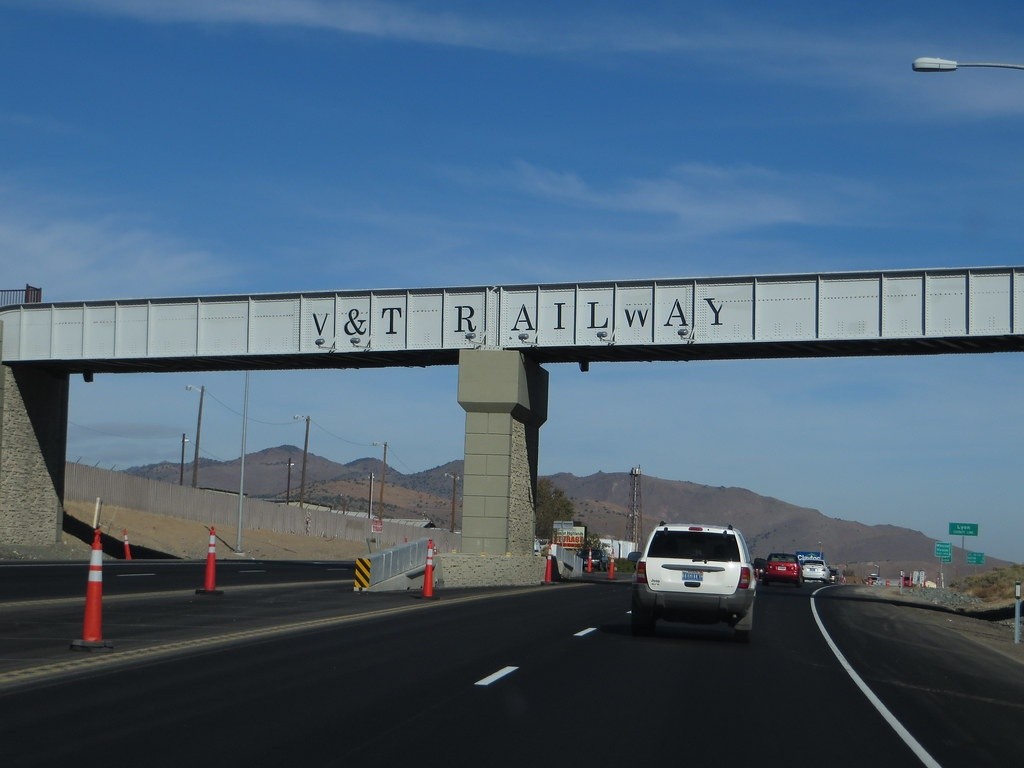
[828,568,839,584]
[577,548,609,571]
[800,559,831,584]
[754,553,804,586]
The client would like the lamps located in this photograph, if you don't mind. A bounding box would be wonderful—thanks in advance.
[596,331,615,344]
[315,339,337,351]
[678,328,696,343]
[519,333,539,346]
[350,338,370,349]
[465,332,483,346]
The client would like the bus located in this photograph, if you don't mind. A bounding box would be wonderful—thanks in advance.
[867,575,881,586]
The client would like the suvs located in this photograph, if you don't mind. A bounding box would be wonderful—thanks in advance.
[628,521,766,642]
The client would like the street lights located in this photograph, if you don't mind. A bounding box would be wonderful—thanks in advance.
[818,541,822,559]
